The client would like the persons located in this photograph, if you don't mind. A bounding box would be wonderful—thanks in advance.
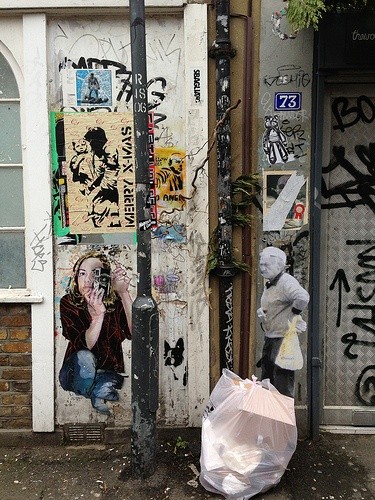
[257,246,310,401]
[87,73,100,99]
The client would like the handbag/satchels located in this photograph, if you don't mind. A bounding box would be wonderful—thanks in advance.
[275,316,303,370]
[198,369,298,500]
